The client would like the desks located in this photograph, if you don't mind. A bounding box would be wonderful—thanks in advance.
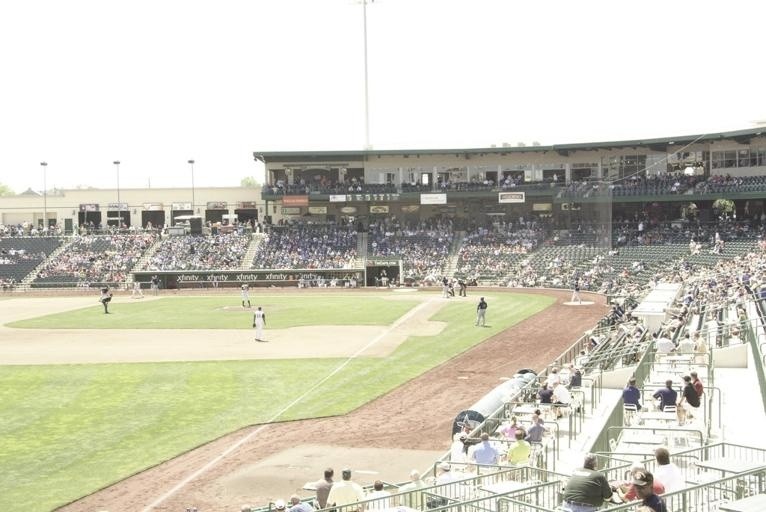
[511,405,543,422]
[653,354,694,384]
[623,432,665,445]
[718,494,766,511]
[696,456,765,474]
[479,480,544,499]
[640,411,678,424]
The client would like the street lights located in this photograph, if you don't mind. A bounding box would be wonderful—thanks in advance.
[40,162,50,231]
[113,160,122,229]
[187,159,195,215]
[354,0,375,148]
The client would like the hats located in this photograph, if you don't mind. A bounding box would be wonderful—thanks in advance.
[631,469,653,485]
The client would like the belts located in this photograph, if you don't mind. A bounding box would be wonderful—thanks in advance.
[565,499,595,507]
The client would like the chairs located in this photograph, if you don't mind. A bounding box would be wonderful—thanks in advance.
[664,404,678,413]
[0,237,139,289]
[610,438,617,452]
[623,403,638,425]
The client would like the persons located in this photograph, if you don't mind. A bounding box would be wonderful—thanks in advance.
[442,278,454,296]
[563,454,613,510]
[474,301,487,326]
[676,376,700,425]
[459,210,765,296]
[254,214,456,288]
[653,448,682,492]
[614,462,666,510]
[500,409,547,444]
[240,281,253,308]
[2,220,162,290]
[145,218,253,272]
[240,461,459,512]
[436,173,522,190]
[565,174,744,195]
[150,275,161,292]
[691,372,703,397]
[270,175,363,194]
[538,363,582,418]
[578,301,746,369]
[653,380,677,412]
[471,432,500,470]
[132,280,142,296]
[457,277,467,296]
[100,292,114,313]
[251,307,269,344]
[622,378,642,411]
[507,429,530,478]
